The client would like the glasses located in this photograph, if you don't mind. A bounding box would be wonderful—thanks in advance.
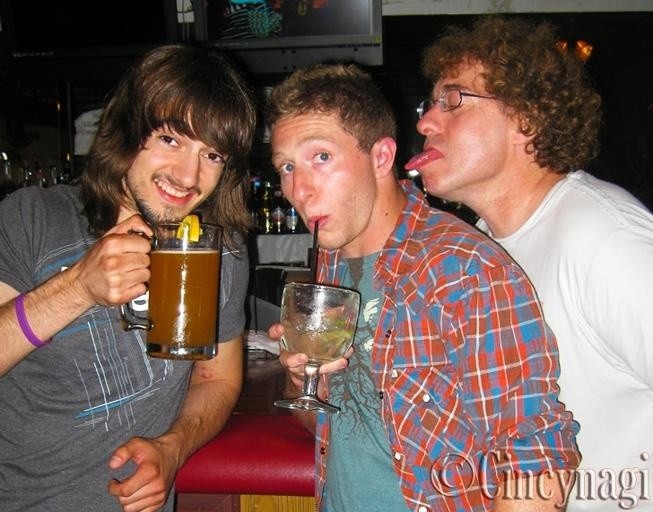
[422,84,501,113]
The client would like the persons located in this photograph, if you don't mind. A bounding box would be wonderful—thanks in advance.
[400,20,651,511]
[263,62,582,510]
[0,45,256,510]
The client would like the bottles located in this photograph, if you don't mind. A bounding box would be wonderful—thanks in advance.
[243,179,300,234]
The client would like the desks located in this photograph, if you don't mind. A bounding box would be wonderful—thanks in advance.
[174,412,319,512]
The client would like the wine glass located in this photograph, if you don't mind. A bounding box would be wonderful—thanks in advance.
[273,282,361,414]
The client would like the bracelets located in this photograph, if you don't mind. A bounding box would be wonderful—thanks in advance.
[14,291,52,346]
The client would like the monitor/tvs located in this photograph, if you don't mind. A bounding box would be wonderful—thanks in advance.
[192,0,383,50]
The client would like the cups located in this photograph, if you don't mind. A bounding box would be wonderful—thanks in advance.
[118,220,224,361]
